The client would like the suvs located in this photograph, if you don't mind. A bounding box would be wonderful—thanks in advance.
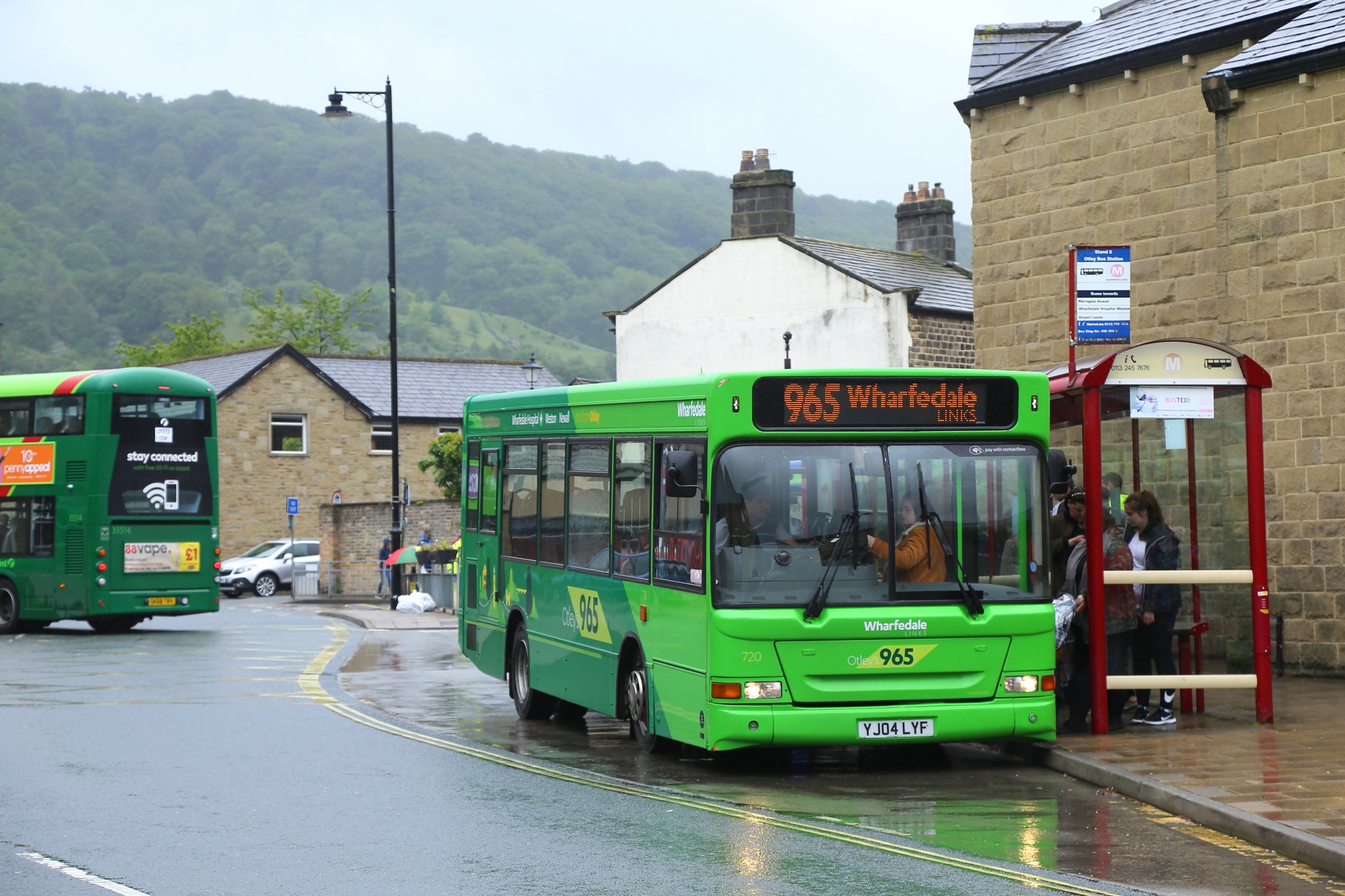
[220,537,320,598]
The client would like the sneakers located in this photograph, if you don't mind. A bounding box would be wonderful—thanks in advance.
[1143,705,1177,725]
[1130,705,1151,724]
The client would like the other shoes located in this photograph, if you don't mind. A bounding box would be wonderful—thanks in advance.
[1056,720,1088,734]
[375,595,382,599]
[1109,716,1124,731]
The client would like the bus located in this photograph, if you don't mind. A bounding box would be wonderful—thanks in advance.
[0,366,222,636]
[457,367,1078,757]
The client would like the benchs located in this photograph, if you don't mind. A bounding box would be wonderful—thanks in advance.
[1165,616,1211,714]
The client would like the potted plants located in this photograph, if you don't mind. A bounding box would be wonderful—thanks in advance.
[414,544,430,565]
[430,542,458,562]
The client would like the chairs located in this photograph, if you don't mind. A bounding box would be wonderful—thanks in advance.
[8,414,81,435]
[510,512,970,583]
[3,515,52,553]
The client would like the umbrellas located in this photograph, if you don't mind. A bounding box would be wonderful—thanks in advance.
[382,544,428,565]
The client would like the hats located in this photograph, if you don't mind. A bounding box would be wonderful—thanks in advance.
[423,528,431,534]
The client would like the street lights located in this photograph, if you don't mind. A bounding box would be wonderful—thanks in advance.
[319,76,402,611]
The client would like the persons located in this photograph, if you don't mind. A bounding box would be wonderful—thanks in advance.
[417,529,434,569]
[865,488,948,583]
[714,478,823,562]
[376,539,393,600]
[977,472,1180,734]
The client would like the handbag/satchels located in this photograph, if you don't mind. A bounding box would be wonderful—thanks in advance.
[1053,593,1077,650]
